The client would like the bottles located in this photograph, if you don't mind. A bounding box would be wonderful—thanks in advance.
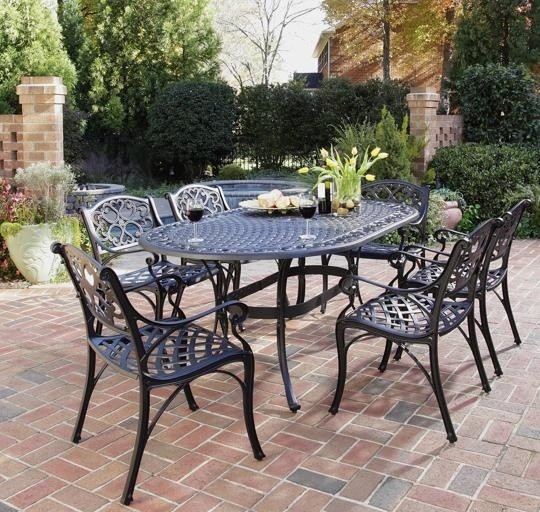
[318,161,331,214]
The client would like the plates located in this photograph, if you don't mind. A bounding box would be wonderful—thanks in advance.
[239,200,313,214]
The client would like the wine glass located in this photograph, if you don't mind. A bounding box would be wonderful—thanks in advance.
[186,198,204,243]
[298,195,316,239]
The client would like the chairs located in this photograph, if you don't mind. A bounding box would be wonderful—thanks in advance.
[388,197,532,377]
[320,177,429,313]
[326,215,503,443]
[81,195,228,359]
[162,183,266,331]
[51,240,267,505]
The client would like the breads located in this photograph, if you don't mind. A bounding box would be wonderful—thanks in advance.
[275,195,290,208]
[268,189,282,206]
[257,194,274,208]
[289,196,312,207]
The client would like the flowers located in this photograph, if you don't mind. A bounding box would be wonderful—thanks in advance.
[298,145,389,202]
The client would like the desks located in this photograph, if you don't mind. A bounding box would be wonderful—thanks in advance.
[136,202,418,414]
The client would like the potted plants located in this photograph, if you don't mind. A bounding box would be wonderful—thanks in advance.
[0,160,81,285]
